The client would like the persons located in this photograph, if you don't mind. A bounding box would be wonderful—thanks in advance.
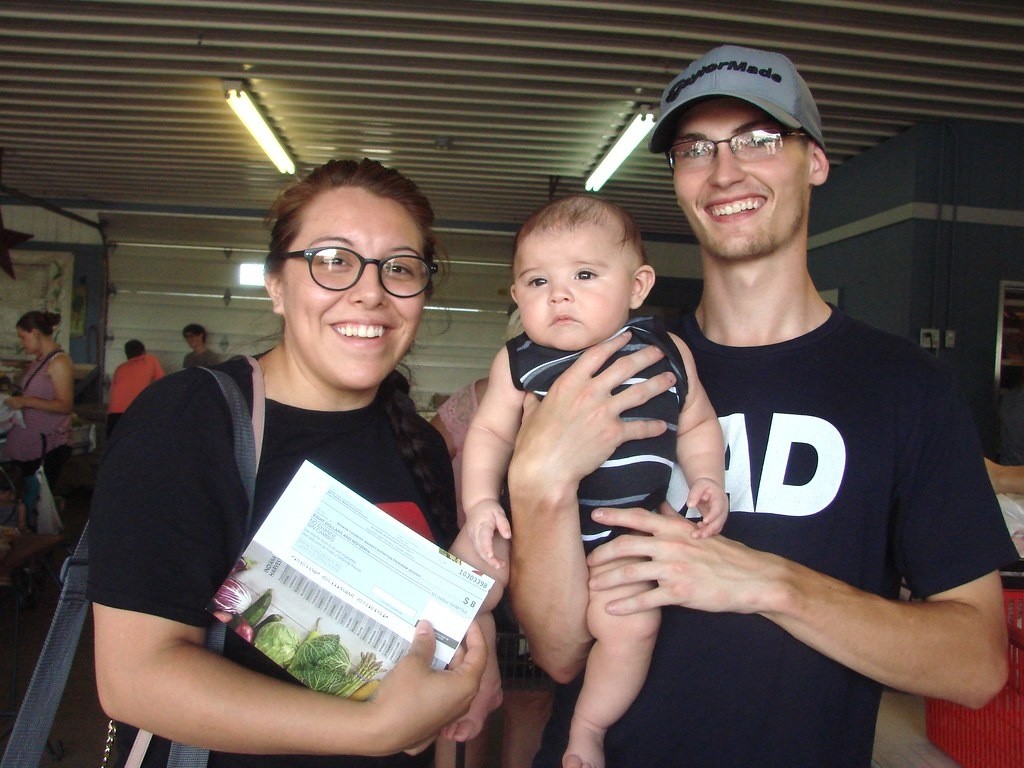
[179,323,222,369]
[11,309,77,533]
[82,158,512,768]
[439,195,733,768]
[508,53,1024,768]
[106,340,167,437]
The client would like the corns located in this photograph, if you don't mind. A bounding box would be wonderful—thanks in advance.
[348,679,382,702]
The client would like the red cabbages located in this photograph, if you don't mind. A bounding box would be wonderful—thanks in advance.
[207,576,252,615]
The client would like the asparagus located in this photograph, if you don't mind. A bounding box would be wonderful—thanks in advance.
[332,651,387,699]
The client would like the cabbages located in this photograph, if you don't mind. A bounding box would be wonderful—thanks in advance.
[253,621,352,694]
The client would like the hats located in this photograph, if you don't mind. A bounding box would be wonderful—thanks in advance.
[655,42,823,158]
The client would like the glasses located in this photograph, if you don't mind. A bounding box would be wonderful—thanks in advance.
[664,129,810,172]
[184,333,199,340]
[280,241,442,303]
